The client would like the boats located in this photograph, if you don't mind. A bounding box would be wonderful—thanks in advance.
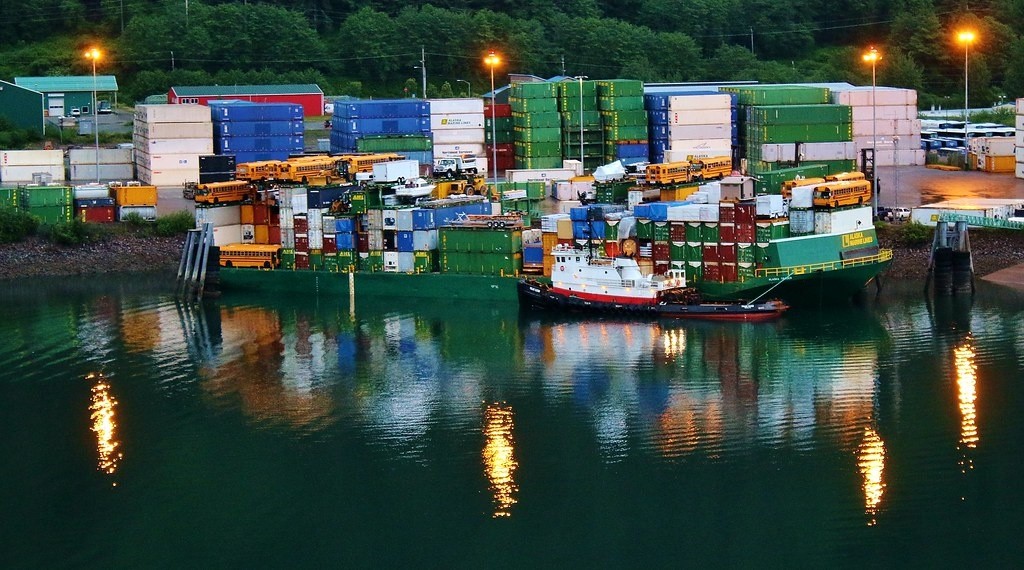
[516,206,790,323]
[189,155,894,307]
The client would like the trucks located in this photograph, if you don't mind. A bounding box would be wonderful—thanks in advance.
[433,156,478,178]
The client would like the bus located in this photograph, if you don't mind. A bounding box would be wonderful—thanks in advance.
[236,153,405,185]
[97,101,111,114]
[689,156,732,182]
[219,243,282,268]
[812,179,872,207]
[195,180,252,205]
[823,172,865,183]
[781,178,825,198]
[645,162,690,186]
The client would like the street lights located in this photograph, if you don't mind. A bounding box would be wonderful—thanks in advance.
[485,54,497,193]
[959,32,973,171]
[85,49,100,183]
[863,49,883,222]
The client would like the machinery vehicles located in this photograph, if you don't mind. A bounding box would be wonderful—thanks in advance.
[447,176,487,196]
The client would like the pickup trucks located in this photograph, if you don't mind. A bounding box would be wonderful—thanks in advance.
[888,207,911,221]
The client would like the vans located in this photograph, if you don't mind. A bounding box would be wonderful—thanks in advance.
[70,109,80,117]
